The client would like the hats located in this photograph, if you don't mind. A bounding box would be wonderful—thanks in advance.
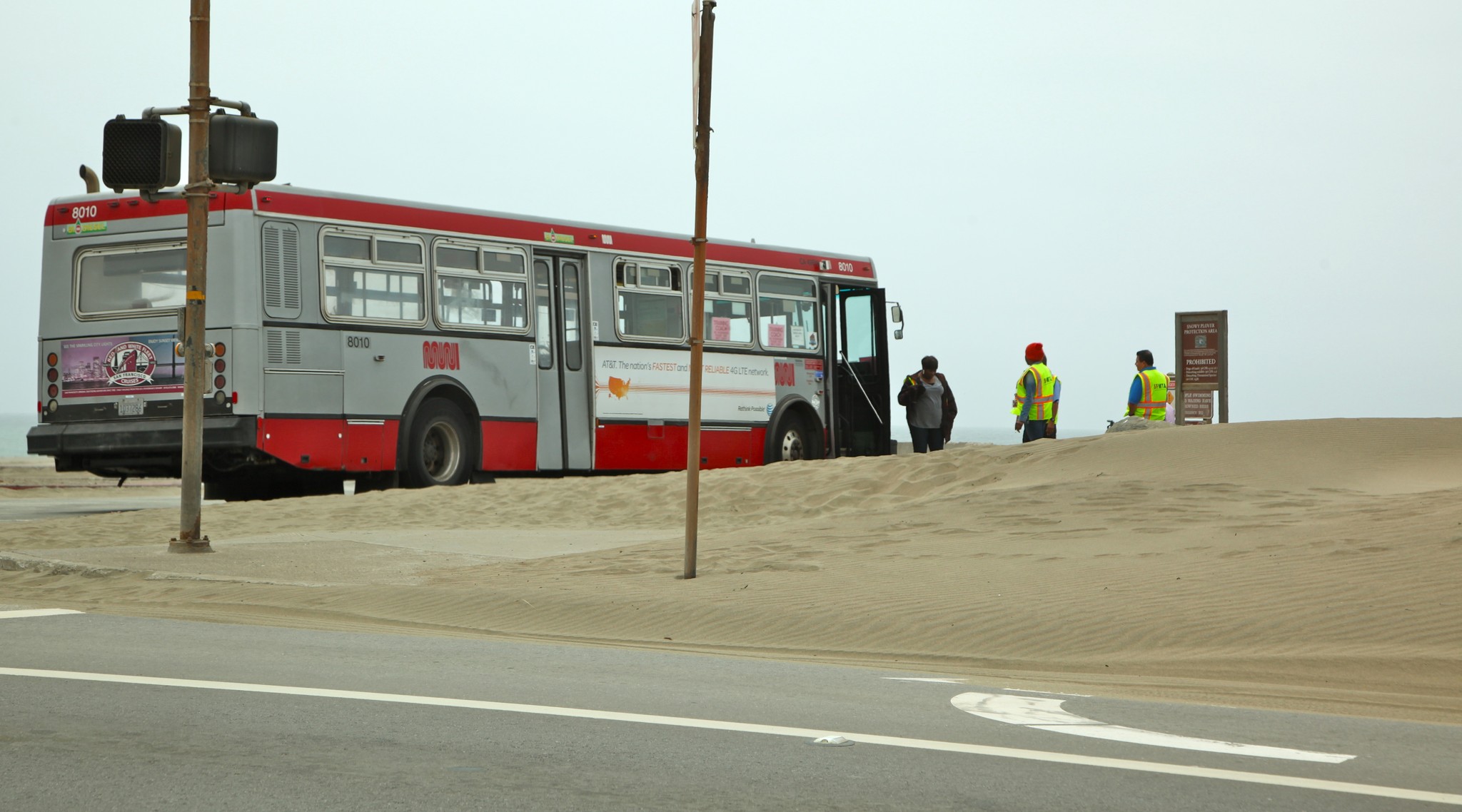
[1025,343,1044,361]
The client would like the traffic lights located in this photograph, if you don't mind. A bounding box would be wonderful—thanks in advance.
[102,115,182,194]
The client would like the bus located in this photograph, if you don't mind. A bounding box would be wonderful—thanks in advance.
[24,165,905,502]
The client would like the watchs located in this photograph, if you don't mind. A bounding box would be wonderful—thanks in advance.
[1050,418,1055,422]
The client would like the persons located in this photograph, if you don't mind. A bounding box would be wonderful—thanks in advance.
[1124,350,1175,426]
[898,355,958,453]
[1012,343,1060,444]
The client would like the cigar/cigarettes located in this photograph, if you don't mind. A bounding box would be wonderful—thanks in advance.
[1015,429,1017,433]
